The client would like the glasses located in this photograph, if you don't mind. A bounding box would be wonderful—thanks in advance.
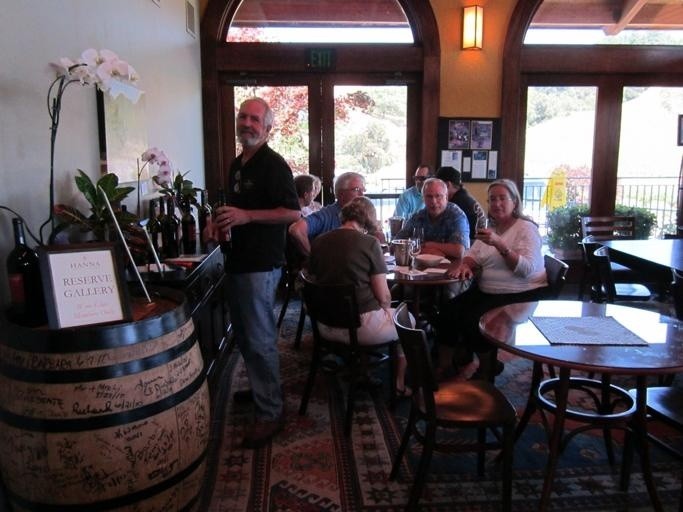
[413,176,425,180]
[343,187,360,192]
[233,170,241,193]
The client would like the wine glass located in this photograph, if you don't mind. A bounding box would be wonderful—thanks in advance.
[407,237,422,274]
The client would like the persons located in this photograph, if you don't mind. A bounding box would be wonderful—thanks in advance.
[448,180,548,383]
[282,163,487,402]
[203,96,301,452]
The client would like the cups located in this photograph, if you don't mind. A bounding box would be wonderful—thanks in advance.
[413,227,425,242]
[474,216,488,233]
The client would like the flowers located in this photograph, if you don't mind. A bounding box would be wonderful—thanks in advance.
[44,46,144,240]
[134,147,204,224]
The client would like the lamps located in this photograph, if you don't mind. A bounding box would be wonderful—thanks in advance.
[461,5,483,48]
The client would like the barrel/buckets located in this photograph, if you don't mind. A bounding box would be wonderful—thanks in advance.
[392,240,416,266]
[0,279,209,512]
[389,217,404,234]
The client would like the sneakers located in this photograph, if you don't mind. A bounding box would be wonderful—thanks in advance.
[234,389,254,403]
[470,361,504,384]
[242,424,277,446]
[443,353,472,377]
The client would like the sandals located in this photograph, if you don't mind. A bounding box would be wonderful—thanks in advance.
[395,386,414,400]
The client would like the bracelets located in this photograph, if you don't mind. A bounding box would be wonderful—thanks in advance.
[500,247,512,257]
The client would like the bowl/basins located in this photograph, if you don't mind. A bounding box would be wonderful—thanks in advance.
[416,254,444,266]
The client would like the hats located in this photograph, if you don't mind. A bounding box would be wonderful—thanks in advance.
[428,166,460,181]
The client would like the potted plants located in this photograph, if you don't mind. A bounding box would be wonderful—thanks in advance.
[542,204,589,259]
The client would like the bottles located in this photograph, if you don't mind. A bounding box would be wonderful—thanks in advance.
[146,181,233,263]
[6,218,48,329]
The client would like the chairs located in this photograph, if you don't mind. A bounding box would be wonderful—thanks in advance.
[577,209,636,281]
[287,237,473,350]
[622,385,680,497]
[387,303,516,506]
[296,270,393,432]
[579,233,648,304]
[593,243,670,316]
[544,255,568,374]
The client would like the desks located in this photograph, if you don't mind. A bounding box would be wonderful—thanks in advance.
[600,238,682,308]
[479,300,683,509]
